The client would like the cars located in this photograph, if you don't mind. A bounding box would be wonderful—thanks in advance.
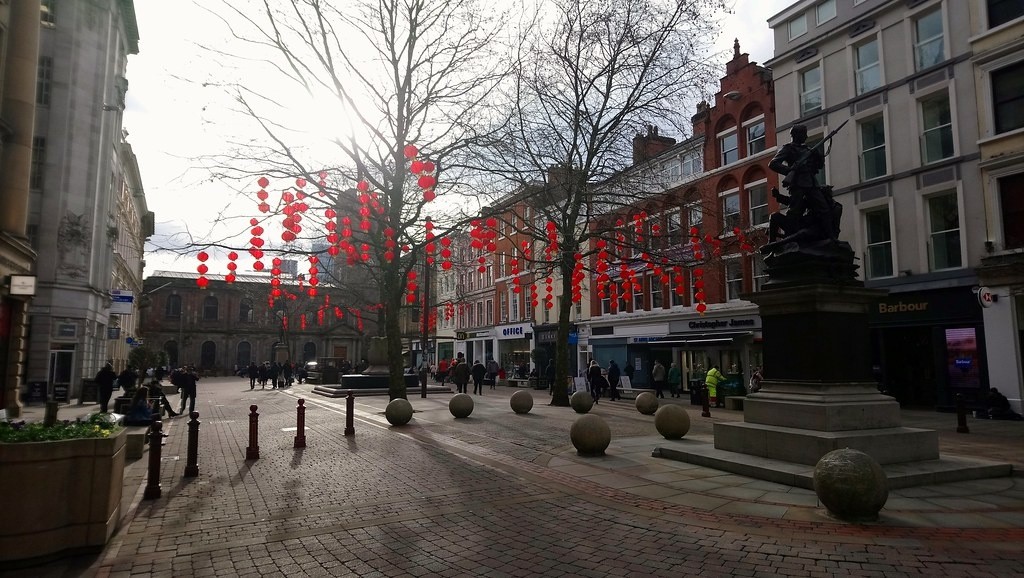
[135,367,156,378]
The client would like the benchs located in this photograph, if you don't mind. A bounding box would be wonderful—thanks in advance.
[126,425,149,459]
[603,387,657,398]
[508,378,528,387]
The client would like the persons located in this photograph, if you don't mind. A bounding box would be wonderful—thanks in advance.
[95,362,200,446]
[759,124,843,254]
[234,358,366,391]
[585,358,763,409]
[407,357,527,396]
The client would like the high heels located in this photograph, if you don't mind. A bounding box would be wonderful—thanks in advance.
[251,386,254,389]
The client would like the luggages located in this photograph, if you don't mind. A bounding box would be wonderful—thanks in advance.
[279,378,284,388]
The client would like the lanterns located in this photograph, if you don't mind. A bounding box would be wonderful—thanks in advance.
[198,145,751,333]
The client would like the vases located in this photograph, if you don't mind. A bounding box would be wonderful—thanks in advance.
[0,427,128,562]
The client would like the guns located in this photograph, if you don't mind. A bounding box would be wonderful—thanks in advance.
[781,118,849,186]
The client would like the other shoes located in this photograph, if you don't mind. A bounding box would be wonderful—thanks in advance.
[660,396,664,399]
[289,383,292,386]
[169,413,180,418]
[671,395,674,397]
[479,393,482,395]
[273,385,277,389]
[610,398,616,401]
[473,390,476,395]
[709,403,717,408]
[656,395,659,398]
[676,395,680,398]
[618,396,622,400]
[592,399,599,404]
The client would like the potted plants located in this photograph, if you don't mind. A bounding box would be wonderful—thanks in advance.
[114,345,162,422]
[529,348,548,389]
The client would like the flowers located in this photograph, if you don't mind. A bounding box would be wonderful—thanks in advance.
[0,412,123,441]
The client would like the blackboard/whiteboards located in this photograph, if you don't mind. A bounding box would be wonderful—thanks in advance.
[79,378,100,402]
[51,382,70,402]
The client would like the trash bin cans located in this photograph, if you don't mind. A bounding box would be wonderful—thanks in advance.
[698,378,706,384]
[690,379,701,405]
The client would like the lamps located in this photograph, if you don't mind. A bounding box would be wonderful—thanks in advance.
[984,241,994,253]
